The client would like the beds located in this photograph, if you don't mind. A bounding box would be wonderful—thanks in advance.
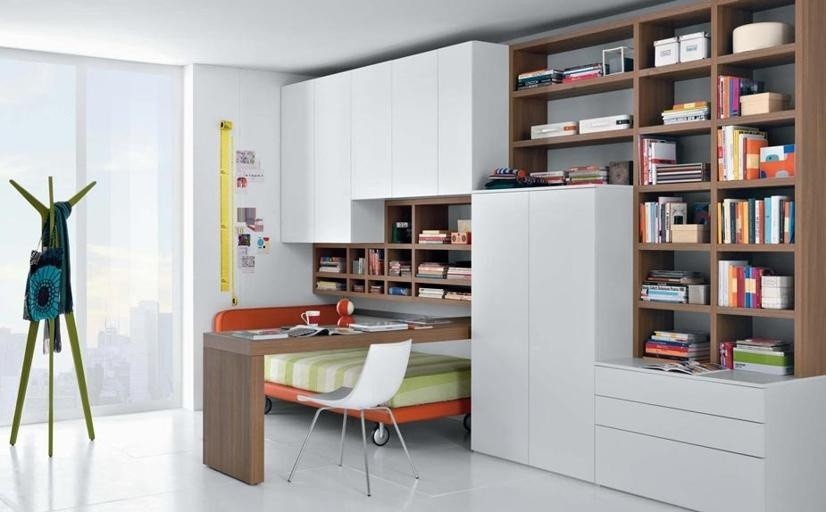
[210,301,470,446]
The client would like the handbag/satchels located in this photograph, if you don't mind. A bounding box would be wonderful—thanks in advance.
[23,247,64,321]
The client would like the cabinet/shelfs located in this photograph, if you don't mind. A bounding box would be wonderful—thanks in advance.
[509,1,826,379]
[310,198,470,303]
[596,357,826,510]
[470,184,632,493]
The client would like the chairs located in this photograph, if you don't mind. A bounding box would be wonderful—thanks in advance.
[287,337,420,497]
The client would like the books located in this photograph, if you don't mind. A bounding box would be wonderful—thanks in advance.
[638,101,729,378]
[717,76,796,352]
[229,315,454,341]
[494,57,636,186]
[314,214,471,303]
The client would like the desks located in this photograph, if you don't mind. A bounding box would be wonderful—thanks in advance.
[200,312,470,485]
